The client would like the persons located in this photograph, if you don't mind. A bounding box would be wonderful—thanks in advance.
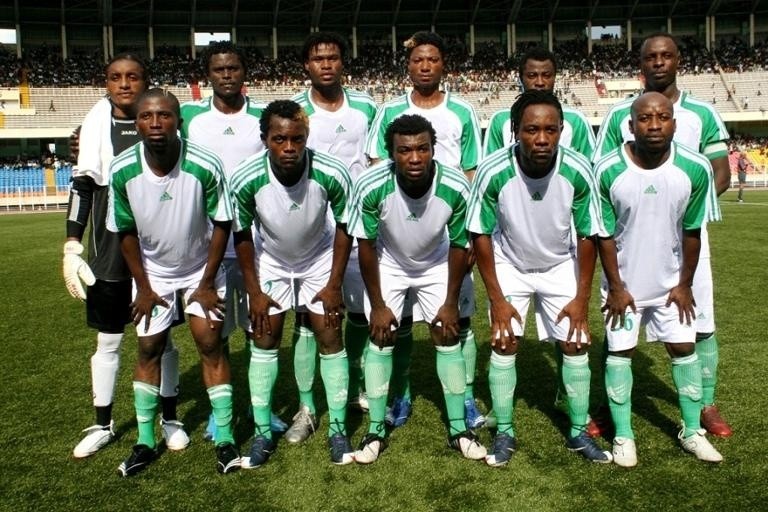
[276,31,398,444]
[345,34,410,87]
[364,31,487,430]
[0,42,106,87]
[345,114,487,464]
[147,43,209,89]
[594,90,722,465]
[178,41,289,442]
[228,100,355,469]
[448,34,520,99]
[0,151,74,169]
[242,29,305,87]
[63,55,191,459]
[105,88,242,478]
[737,153,748,203]
[466,89,612,466]
[482,47,605,439]
[675,33,768,73]
[588,36,732,438]
[728,133,768,158]
[556,34,642,91]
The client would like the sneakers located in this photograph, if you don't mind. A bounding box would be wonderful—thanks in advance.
[554,390,593,425]
[327,419,354,467]
[116,441,158,479]
[586,413,611,435]
[487,423,515,468]
[674,421,724,461]
[285,405,317,443]
[568,425,613,464]
[484,404,514,428]
[205,413,241,442]
[464,398,485,429]
[354,421,387,464]
[159,413,190,450]
[448,418,488,461]
[701,404,731,435]
[251,404,288,432]
[353,394,371,413]
[388,393,411,430]
[72,419,116,460]
[213,432,241,478]
[611,434,638,466]
[242,424,275,471]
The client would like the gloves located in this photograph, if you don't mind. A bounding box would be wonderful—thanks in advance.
[61,241,96,302]
